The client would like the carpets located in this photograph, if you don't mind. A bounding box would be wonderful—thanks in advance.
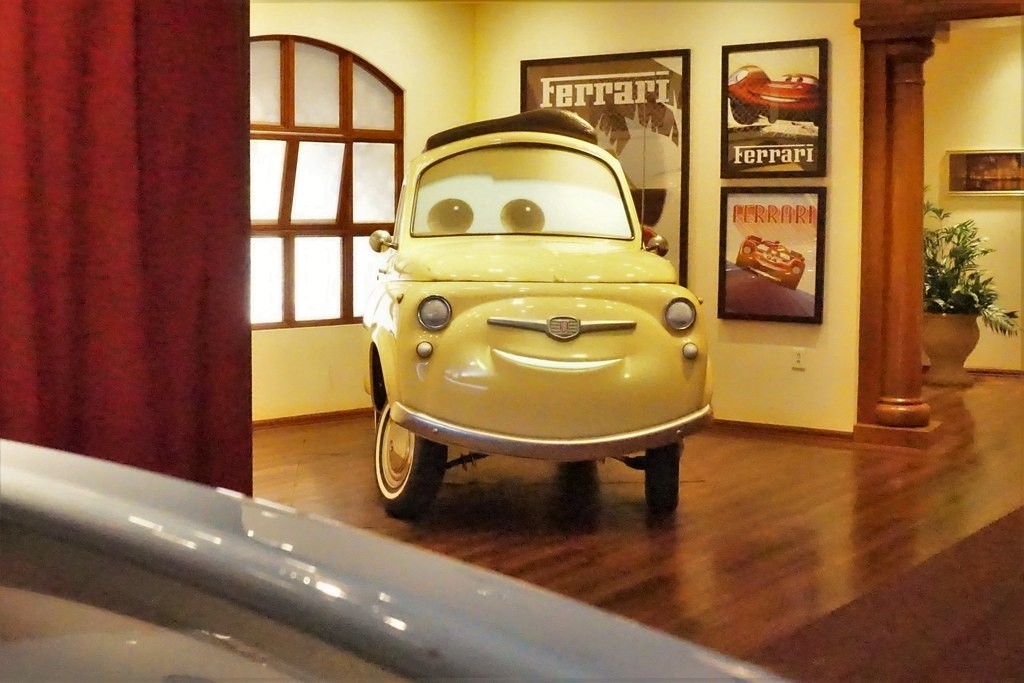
[739,505,1024,683]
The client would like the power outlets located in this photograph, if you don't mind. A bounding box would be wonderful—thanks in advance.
[791,346,805,369]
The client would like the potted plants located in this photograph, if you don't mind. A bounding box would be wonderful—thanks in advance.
[922,187,1020,385]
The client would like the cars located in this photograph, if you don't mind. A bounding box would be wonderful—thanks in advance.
[359,108,714,519]
[736,236,805,292]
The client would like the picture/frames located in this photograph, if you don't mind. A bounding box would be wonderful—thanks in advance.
[717,185,826,324]
[521,48,693,286]
[720,38,830,180]
[946,149,1024,197]
